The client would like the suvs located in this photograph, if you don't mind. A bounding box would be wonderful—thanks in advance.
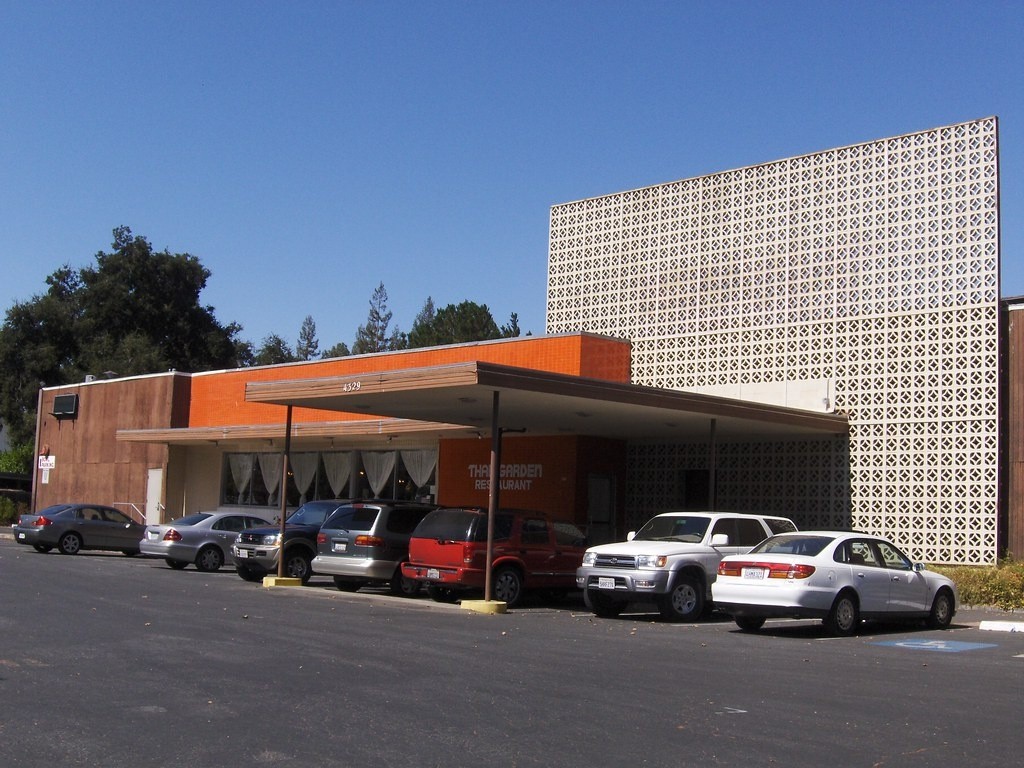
[401,504,597,611]
[231,497,384,586]
[309,498,448,600]
[574,512,802,622]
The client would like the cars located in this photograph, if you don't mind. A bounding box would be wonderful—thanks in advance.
[138,511,276,571]
[12,503,148,557]
[709,529,960,637]
